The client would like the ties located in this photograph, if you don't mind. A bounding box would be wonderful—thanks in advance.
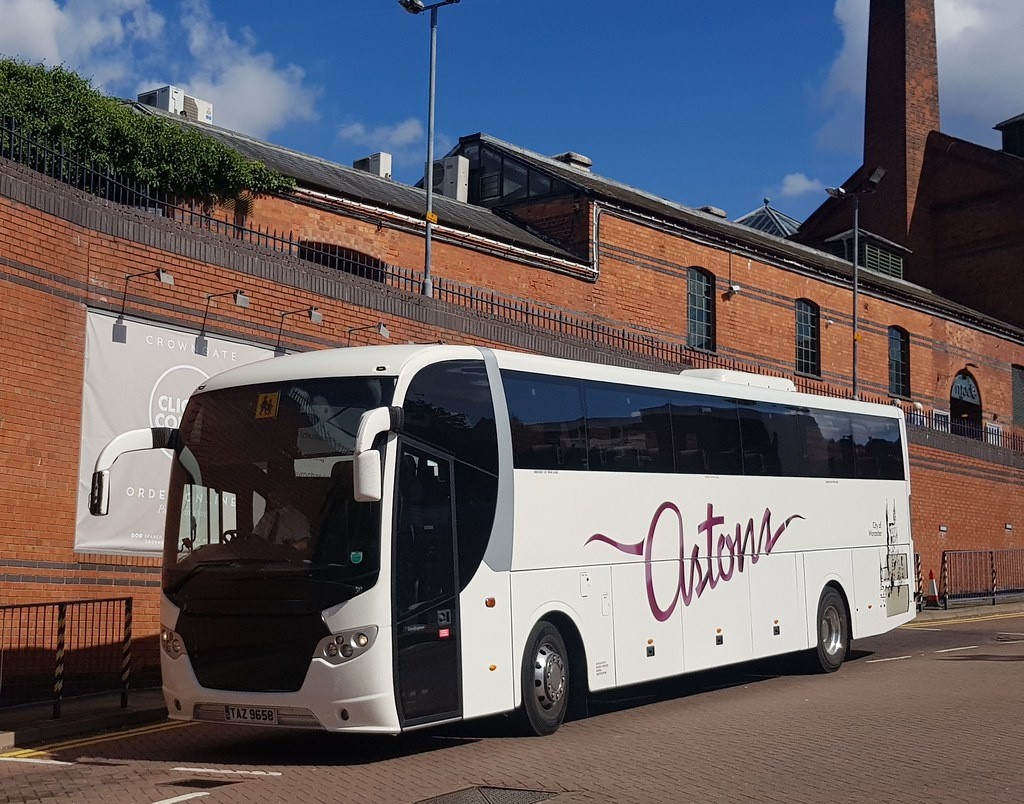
[267,512,280,544]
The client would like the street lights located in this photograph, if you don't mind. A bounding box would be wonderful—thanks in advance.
[826,186,876,400]
[398,0,463,297]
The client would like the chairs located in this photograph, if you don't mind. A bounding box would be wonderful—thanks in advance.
[265,476,331,559]
[331,460,370,524]
[402,456,415,490]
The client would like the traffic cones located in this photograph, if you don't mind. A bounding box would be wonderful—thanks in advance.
[923,569,945,610]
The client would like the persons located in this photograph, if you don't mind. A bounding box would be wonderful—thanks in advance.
[254,476,312,552]
[891,398,926,426]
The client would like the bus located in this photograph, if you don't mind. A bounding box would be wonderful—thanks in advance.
[89,343,917,736]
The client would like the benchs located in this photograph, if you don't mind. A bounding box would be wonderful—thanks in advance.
[518,445,764,475]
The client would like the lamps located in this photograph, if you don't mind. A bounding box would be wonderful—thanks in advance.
[409,339,445,345]
[126,267,175,285]
[208,289,250,307]
[280,306,322,322]
[349,322,390,339]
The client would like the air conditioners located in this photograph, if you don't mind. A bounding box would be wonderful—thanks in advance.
[353,151,392,180]
[424,155,469,204]
[138,85,184,116]
[184,94,213,124]
